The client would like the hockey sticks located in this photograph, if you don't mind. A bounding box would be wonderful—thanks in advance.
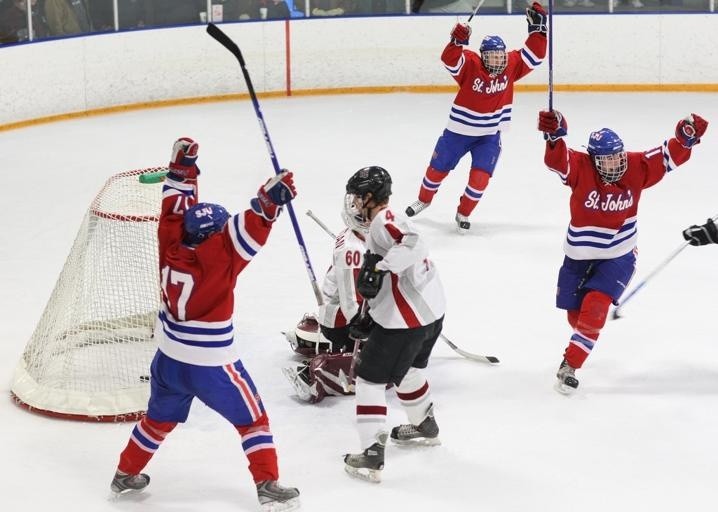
[306,210,500,363]
[207,22,325,306]
[614,216,718,319]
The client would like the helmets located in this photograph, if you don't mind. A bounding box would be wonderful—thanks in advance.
[182,201,231,243]
[586,127,629,183]
[479,34,509,75]
[344,165,392,233]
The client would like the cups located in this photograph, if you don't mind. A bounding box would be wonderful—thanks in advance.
[258,8,268,18]
[199,12,206,23]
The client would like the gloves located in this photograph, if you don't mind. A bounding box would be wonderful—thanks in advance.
[449,21,472,47]
[682,217,718,247]
[674,113,709,149]
[169,136,199,178]
[525,0,549,35]
[257,169,297,221]
[357,252,385,298]
[348,310,373,341]
[537,108,567,142]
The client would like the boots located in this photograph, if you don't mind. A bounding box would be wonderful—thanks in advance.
[556,358,580,389]
[108,471,151,495]
[342,439,386,473]
[255,480,302,505]
[391,403,440,441]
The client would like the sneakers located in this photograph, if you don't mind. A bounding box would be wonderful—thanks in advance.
[404,199,433,218]
[296,360,311,387]
[454,212,470,230]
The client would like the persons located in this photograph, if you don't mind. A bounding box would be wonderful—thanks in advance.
[110,137,300,505]
[282,191,396,405]
[0,0,718,45]
[343,165,446,470]
[405,1,548,228]
[538,109,708,389]
